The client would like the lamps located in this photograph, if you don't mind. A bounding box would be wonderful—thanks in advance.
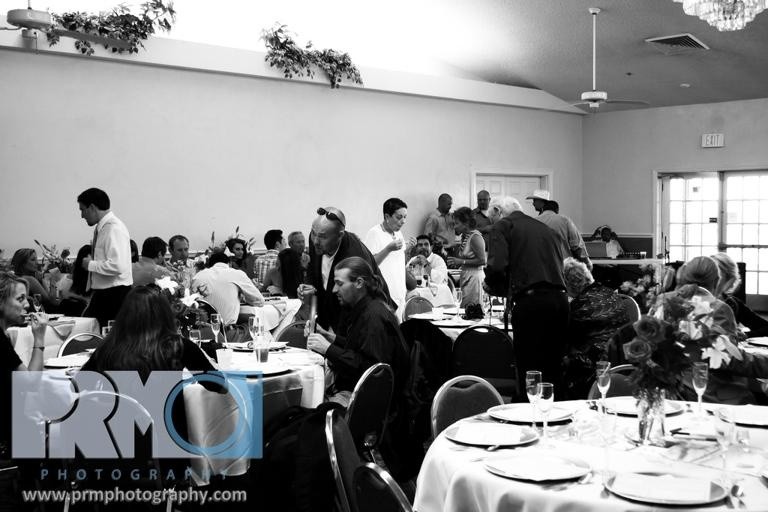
[670,0,767,32]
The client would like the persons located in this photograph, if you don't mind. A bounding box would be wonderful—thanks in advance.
[0,189,767,511]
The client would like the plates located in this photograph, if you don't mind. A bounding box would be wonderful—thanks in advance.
[482,458,592,481]
[597,395,683,416]
[603,471,729,506]
[444,421,540,448]
[745,336,767,346]
[487,401,578,423]
[713,405,768,426]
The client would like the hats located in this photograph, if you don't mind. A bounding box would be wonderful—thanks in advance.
[526,189,550,201]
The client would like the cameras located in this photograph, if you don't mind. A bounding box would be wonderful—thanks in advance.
[462,301,485,320]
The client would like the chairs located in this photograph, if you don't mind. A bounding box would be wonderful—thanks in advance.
[1,231,767,512]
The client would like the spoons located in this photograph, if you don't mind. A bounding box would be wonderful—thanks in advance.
[731,484,746,508]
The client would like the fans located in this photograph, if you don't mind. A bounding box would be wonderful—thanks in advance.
[545,6,648,112]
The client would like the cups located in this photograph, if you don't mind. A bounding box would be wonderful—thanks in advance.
[188,330,201,348]
[432,307,443,321]
[101,320,116,340]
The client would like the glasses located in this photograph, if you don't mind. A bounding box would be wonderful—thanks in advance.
[317,207,346,228]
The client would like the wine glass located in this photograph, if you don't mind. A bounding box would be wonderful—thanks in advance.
[596,358,611,404]
[33,294,41,312]
[249,315,268,363]
[526,370,554,443]
[692,360,709,423]
[210,314,221,343]
[451,287,463,323]
